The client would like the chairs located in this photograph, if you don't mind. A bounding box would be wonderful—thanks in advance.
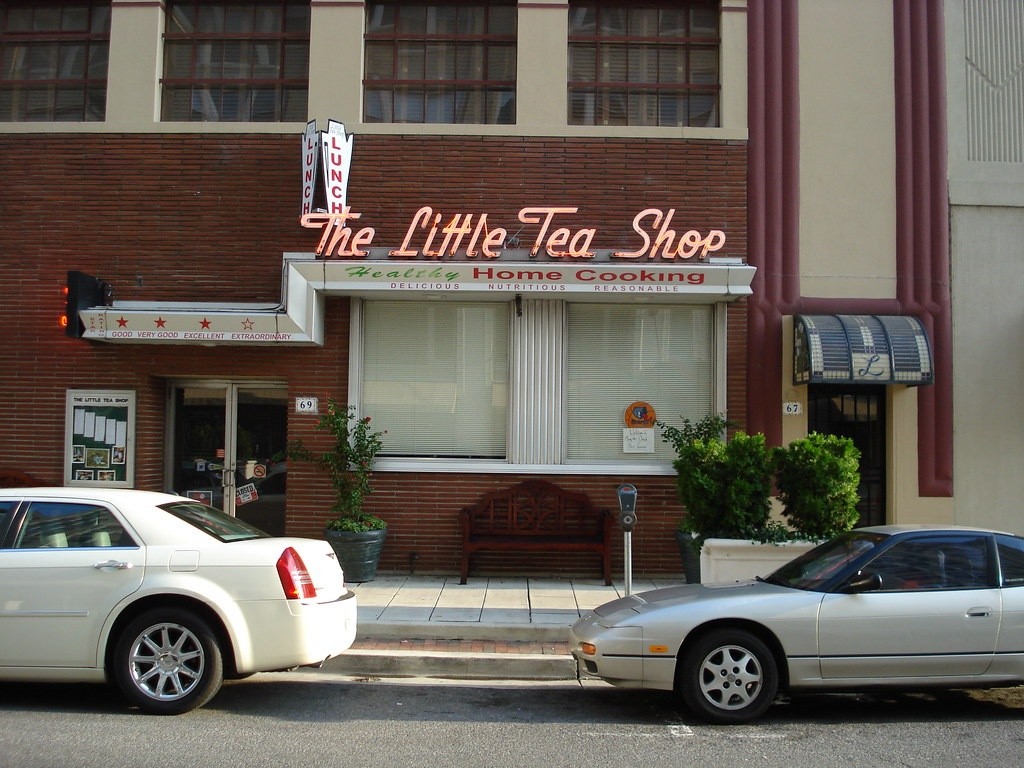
[918,548,948,588]
[44,518,67,548]
[83,518,111,548]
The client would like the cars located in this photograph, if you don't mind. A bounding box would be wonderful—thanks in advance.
[571,522,1024,723]
[0,485,358,716]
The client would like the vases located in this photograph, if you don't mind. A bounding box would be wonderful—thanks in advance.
[323,528,386,581]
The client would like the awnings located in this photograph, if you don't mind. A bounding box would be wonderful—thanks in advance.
[794,314,935,390]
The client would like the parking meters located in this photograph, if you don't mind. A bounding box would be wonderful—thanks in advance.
[616,482,637,600]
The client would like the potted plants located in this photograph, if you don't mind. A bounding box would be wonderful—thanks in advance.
[659,419,861,583]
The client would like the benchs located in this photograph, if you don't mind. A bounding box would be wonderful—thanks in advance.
[458,481,615,585]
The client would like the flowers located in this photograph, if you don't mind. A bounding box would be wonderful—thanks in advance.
[272,392,387,531]
